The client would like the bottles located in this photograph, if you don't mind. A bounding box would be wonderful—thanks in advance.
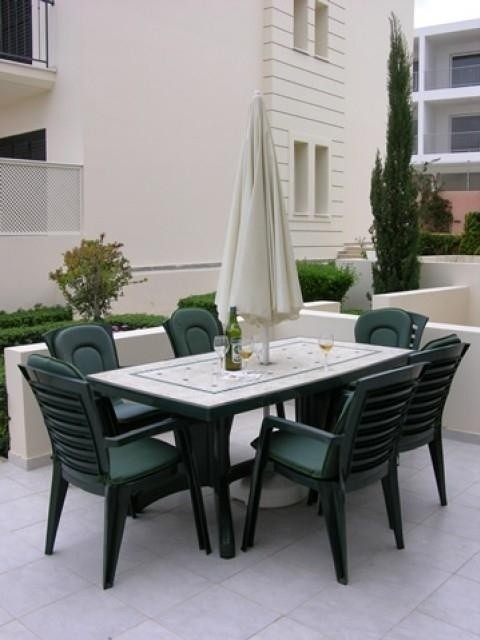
[226,305,243,371]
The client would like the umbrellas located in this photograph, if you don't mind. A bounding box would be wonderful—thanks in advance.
[215,90,305,423]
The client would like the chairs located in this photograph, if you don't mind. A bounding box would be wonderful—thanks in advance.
[241,361,432,585]
[307,334,471,516]
[296,307,430,432]
[163,307,222,357]
[18,353,216,590]
[42,322,171,436]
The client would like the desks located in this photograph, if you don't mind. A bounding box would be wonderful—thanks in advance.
[86,337,417,557]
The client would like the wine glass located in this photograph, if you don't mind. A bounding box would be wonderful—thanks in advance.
[213,335,231,378]
[317,331,337,373]
[236,338,254,379]
